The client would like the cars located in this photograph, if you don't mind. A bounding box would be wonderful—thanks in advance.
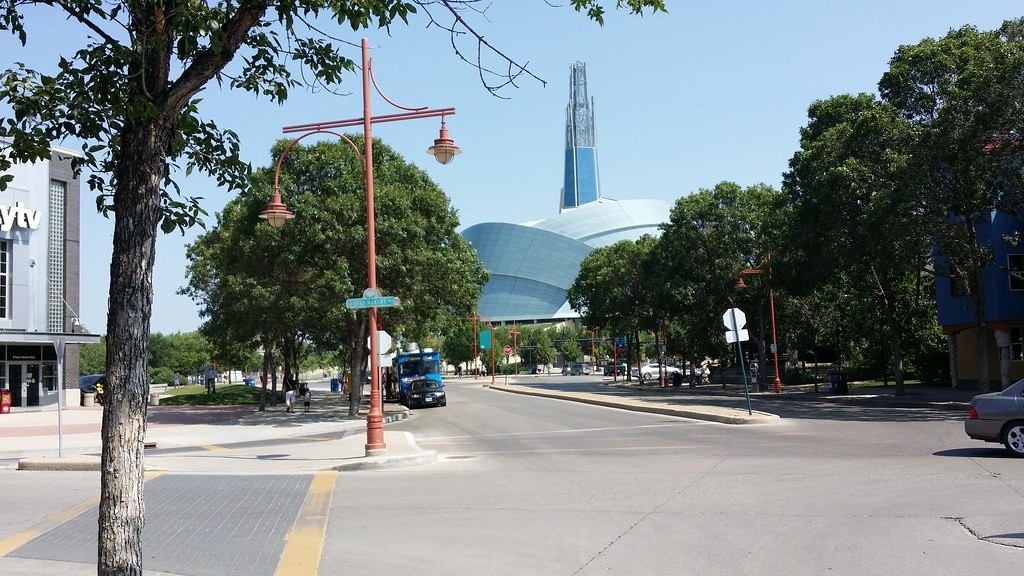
[561,364,592,376]
[404,379,446,409]
[79,374,105,393]
[964,378,1024,456]
[632,363,682,380]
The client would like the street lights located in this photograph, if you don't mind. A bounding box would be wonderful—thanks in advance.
[258,36,463,456]
[458,312,480,379]
[735,242,782,393]
[508,321,521,375]
[582,326,598,373]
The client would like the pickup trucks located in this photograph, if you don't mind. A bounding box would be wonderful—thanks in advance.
[603,360,626,376]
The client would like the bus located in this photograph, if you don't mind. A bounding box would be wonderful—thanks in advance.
[391,352,441,402]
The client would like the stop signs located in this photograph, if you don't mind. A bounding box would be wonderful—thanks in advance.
[504,345,512,353]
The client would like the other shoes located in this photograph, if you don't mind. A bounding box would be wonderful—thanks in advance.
[307,411,309,413]
[304,410,306,412]
[286,407,290,413]
[290,411,294,413]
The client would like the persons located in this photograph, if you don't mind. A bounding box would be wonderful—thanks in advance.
[478,364,487,377]
[283,374,300,414]
[174,372,180,388]
[304,387,312,412]
[458,366,463,379]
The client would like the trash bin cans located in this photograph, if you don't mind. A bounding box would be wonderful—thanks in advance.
[245,376,255,386]
[671,371,683,387]
[330,378,339,392]
[826,361,849,395]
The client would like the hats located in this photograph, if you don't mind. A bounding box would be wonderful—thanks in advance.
[96,384,101,386]
[303,387,309,390]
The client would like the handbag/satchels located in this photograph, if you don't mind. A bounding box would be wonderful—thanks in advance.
[293,389,300,397]
